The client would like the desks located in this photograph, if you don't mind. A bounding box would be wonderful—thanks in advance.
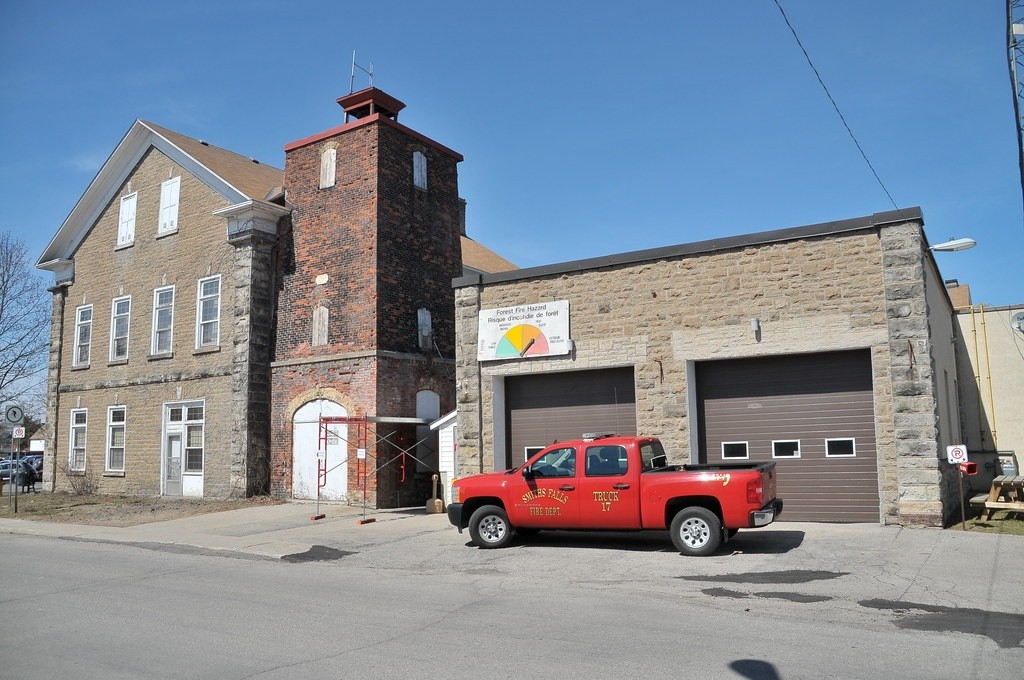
[980,475,1024,522]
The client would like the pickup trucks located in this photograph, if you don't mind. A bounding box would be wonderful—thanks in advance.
[446,432,784,556]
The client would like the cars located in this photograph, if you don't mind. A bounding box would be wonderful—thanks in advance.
[0,453,43,486]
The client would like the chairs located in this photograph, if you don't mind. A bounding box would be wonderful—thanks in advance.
[588,448,616,476]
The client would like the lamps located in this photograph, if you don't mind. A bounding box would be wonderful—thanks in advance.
[568,339,575,352]
[929,237,977,252]
[750,317,758,331]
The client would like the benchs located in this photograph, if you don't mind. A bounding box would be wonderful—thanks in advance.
[984,501,1024,512]
[969,493,989,507]
